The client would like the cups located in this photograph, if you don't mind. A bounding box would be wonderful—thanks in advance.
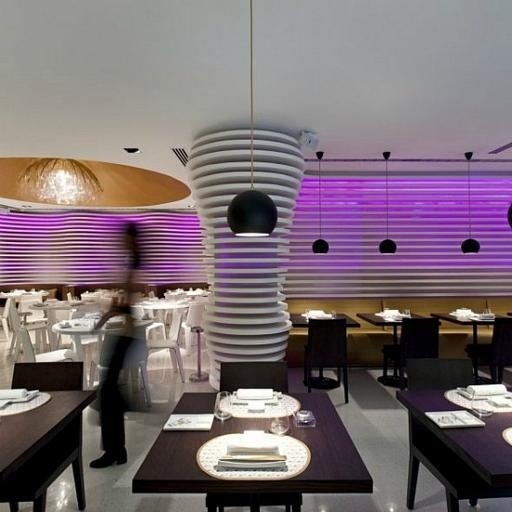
[267,406,291,435]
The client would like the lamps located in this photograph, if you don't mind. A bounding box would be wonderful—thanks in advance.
[18,158,103,205]
[461,152,480,253]
[227,0,278,236]
[380,151,396,253]
[312,151,329,252]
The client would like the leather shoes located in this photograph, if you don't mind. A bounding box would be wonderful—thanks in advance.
[89,449,129,469]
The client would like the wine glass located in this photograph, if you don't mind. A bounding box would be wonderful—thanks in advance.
[471,389,494,422]
[212,391,233,435]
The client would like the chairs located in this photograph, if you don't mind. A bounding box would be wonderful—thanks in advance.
[383,318,438,391]
[406,358,512,512]
[205,362,303,512]
[0,289,205,406]
[0,361,86,512]
[303,318,348,403]
[466,318,512,385]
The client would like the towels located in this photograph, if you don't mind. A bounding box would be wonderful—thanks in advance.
[226,440,282,455]
[466,383,506,396]
[231,388,278,400]
[0,389,27,399]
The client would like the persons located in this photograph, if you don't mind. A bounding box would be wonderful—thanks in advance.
[91,220,141,467]
